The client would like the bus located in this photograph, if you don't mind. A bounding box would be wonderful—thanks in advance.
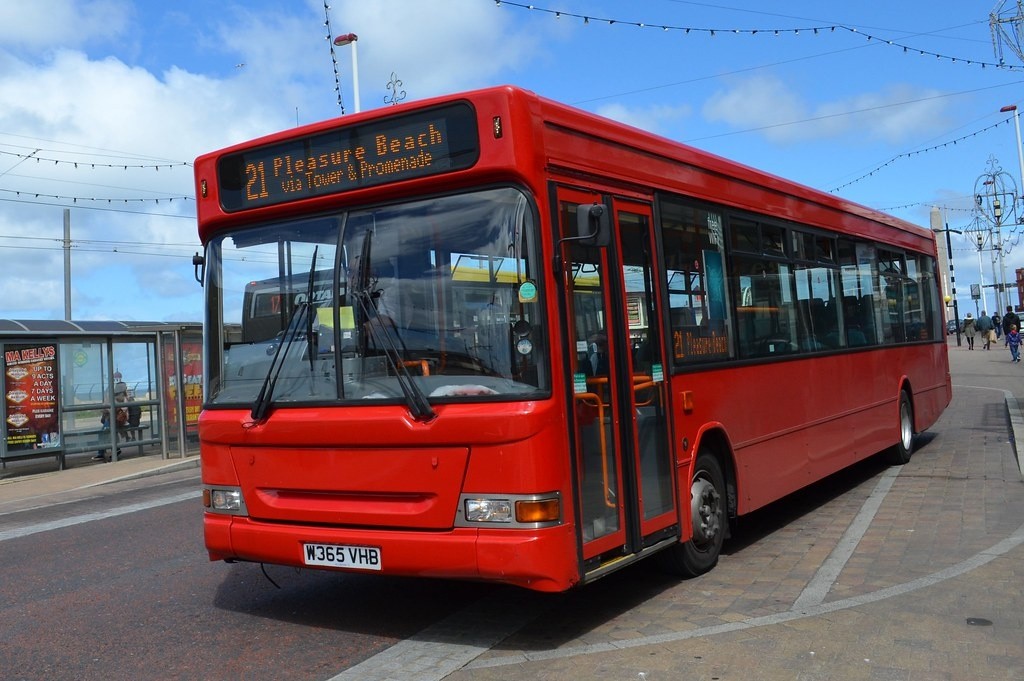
[190,85,954,593]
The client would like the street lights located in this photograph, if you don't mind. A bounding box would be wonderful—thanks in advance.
[1000,104,1024,194]
[334,34,360,114]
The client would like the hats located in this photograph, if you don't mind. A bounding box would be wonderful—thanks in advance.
[967,313,971,317]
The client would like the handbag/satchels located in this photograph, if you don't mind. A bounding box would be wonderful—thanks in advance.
[989,329,997,343]
[115,412,127,426]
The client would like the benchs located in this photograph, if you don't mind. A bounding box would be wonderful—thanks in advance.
[63,424,151,456]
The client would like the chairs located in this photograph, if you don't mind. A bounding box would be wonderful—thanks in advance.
[649,294,880,365]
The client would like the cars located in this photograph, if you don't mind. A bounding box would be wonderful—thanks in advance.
[1017,311,1024,335]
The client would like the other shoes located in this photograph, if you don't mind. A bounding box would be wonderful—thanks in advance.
[1012,359,1016,361]
[126,436,131,442]
[109,449,122,459]
[969,345,974,350]
[131,438,136,441]
[983,343,990,350]
[91,455,105,460]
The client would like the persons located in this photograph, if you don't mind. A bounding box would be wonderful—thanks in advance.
[91,383,141,460]
[310,251,415,334]
[976,311,995,350]
[1003,306,1023,363]
[991,312,1002,341]
[963,313,977,350]
